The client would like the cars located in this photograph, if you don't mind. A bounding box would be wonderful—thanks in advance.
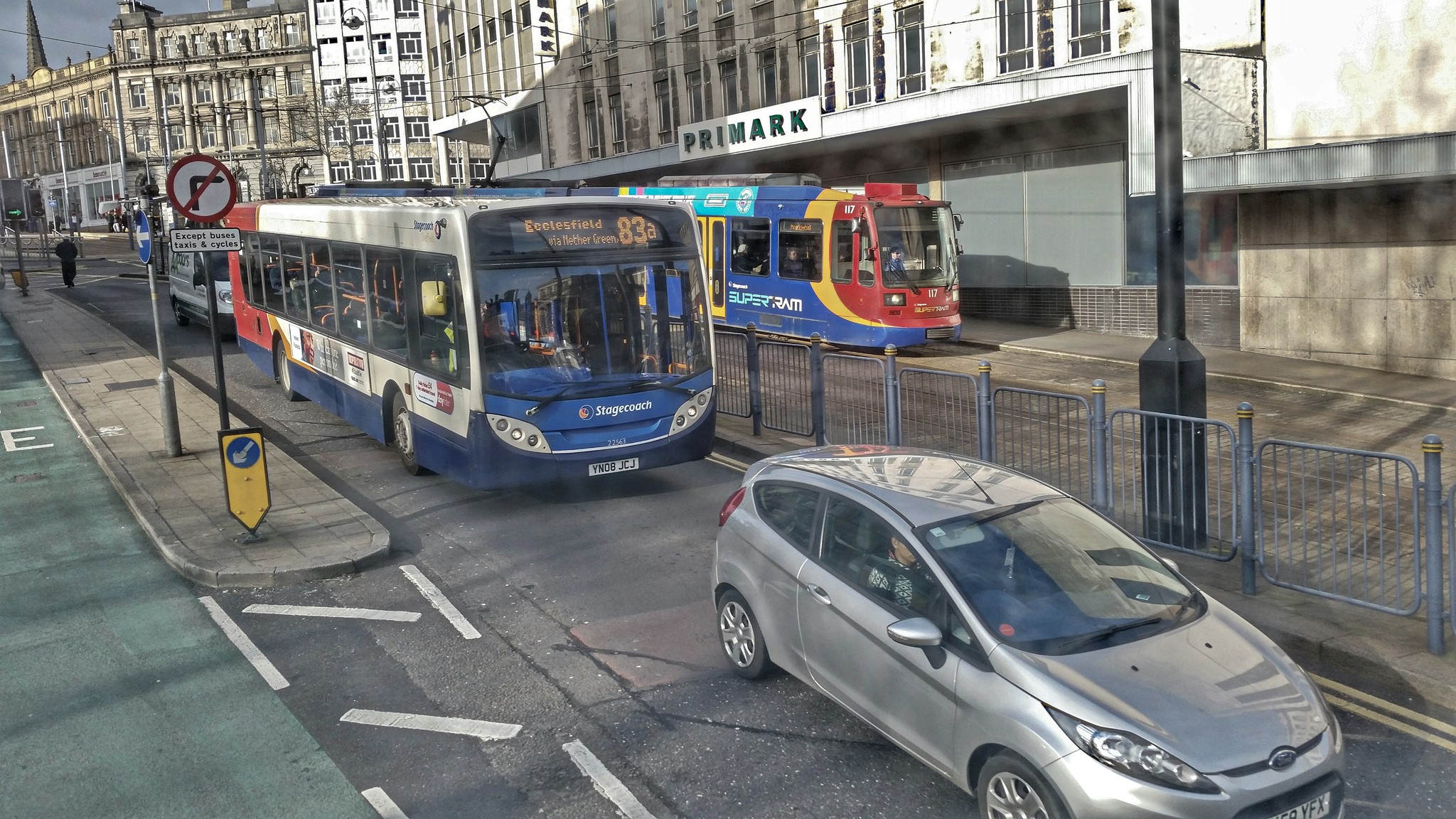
[706,441,1349,819]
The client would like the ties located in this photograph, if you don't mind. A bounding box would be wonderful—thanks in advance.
[896,261,902,273]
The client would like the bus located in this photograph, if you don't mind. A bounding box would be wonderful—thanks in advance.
[311,95,963,349]
[226,192,720,489]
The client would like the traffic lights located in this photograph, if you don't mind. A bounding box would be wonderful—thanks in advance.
[0,180,28,221]
[28,189,47,217]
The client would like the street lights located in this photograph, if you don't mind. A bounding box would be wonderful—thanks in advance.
[98,127,117,213]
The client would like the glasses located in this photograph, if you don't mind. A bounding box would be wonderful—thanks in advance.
[892,252,901,255]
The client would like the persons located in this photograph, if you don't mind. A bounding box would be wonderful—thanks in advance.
[54,213,63,234]
[106,210,129,233]
[871,524,935,620]
[55,236,78,288]
[247,251,402,358]
[732,234,906,281]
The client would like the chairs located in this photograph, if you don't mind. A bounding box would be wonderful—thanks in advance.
[266,261,355,324]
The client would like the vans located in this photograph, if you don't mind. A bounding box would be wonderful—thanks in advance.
[166,242,238,343]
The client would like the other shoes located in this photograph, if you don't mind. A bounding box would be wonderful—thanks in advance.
[67,280,74,287]
